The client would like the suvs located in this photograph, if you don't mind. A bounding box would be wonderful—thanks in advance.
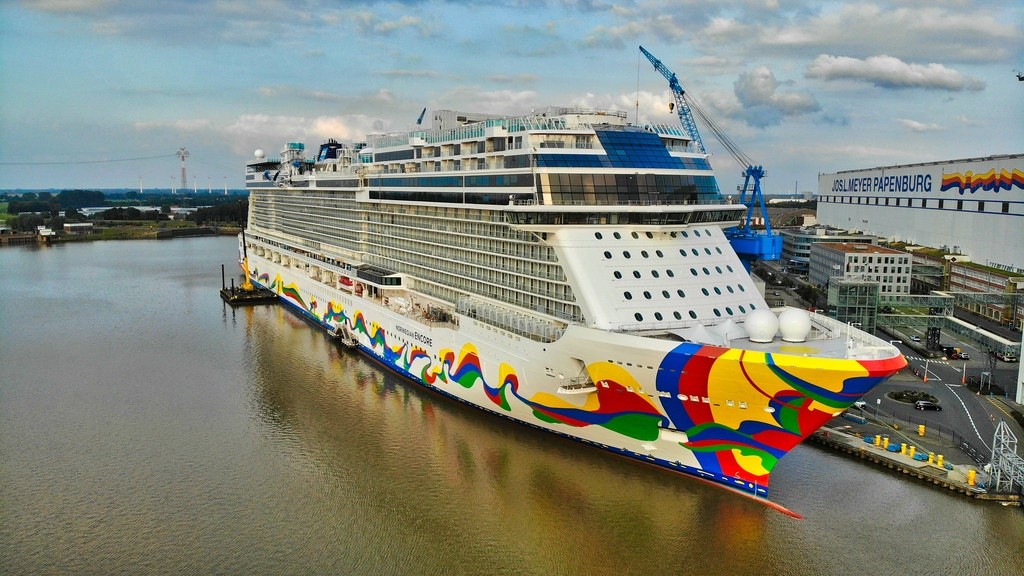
[958,352,969,360]
[915,400,942,412]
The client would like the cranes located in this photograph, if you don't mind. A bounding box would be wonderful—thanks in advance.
[636,44,782,280]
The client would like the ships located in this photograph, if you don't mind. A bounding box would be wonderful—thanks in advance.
[236,107,909,519]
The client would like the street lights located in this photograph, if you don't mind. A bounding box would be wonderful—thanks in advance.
[814,309,824,314]
[851,323,862,327]
[890,340,902,345]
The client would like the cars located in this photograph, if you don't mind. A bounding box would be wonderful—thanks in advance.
[910,334,921,342]
[851,397,867,409]
[773,291,780,296]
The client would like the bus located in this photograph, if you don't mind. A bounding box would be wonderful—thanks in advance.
[990,345,1017,362]
